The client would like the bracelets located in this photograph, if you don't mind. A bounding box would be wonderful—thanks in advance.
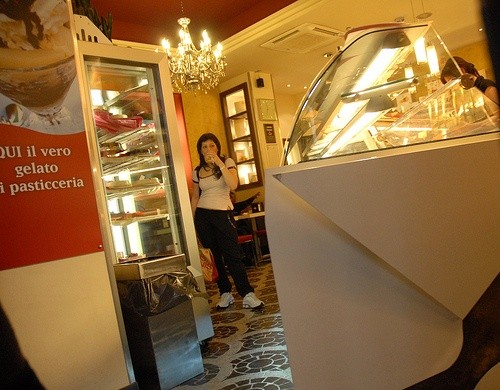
[220,165,225,170]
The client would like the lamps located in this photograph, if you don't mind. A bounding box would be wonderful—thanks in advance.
[404,0,439,92]
[154,0,228,95]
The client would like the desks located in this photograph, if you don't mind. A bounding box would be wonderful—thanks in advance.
[233,212,269,266]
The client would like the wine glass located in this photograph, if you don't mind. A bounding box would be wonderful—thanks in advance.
[0,53,78,127]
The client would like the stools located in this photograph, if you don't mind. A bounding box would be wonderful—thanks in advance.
[257,229,267,254]
[235,235,254,265]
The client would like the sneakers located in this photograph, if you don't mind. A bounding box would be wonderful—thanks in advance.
[243,292,265,308]
[216,292,234,308]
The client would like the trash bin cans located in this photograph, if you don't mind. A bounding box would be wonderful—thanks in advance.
[113,253,204,390]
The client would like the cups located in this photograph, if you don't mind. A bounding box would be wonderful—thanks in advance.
[204,150,214,163]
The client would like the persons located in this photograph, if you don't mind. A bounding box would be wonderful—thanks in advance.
[440,57,500,135]
[190,133,265,309]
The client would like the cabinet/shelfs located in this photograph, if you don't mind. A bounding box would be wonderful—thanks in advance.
[76,38,216,340]
[218,73,285,235]
